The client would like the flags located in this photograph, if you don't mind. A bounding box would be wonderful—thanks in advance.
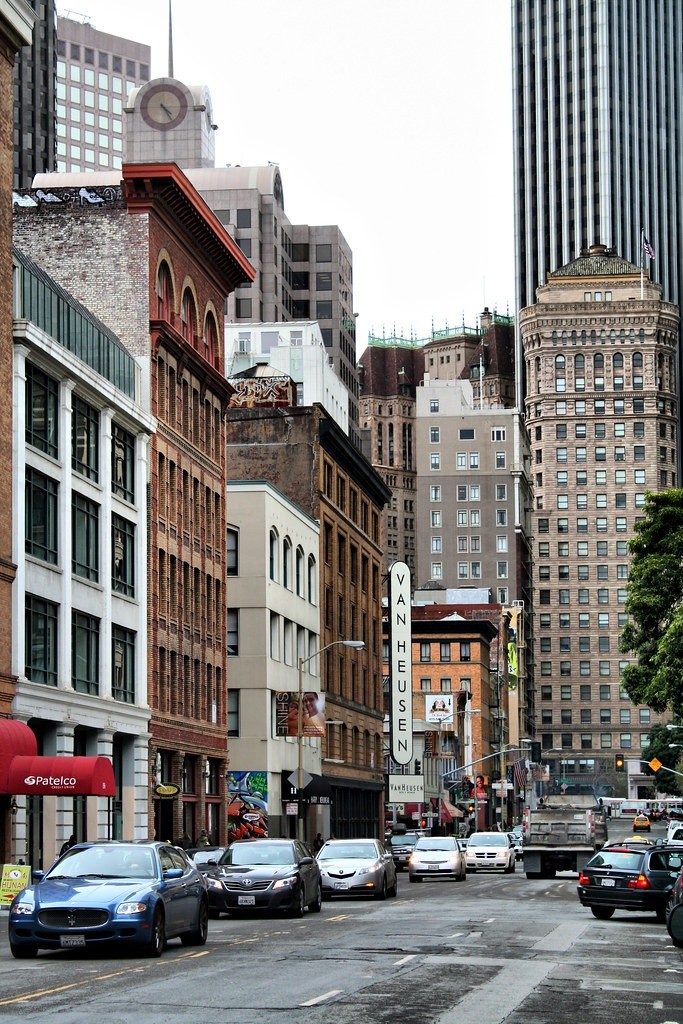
[514,758,528,786]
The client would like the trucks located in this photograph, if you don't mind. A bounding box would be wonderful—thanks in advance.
[522,784,608,879]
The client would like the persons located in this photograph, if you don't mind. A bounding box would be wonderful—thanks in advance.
[59,835,77,857]
[472,776,488,798]
[175,829,211,850]
[288,692,324,736]
[466,819,506,839]
[313,833,336,857]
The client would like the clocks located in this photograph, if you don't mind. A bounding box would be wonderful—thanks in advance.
[139,84,187,131]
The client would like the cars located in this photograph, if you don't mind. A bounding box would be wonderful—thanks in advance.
[462,832,517,874]
[508,825,526,862]
[8,838,209,959]
[203,837,323,919]
[670,863,683,906]
[186,844,230,879]
[632,813,651,833]
[637,807,683,859]
[384,833,423,872]
[316,838,398,902]
[409,837,467,883]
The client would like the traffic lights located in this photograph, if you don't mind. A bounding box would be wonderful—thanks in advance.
[615,754,624,772]
[415,758,421,775]
[422,819,427,827]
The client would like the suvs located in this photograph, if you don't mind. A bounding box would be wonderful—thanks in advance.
[577,842,683,924]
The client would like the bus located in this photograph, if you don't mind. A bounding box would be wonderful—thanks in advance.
[597,797,683,819]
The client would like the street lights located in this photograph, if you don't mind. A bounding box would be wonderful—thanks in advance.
[539,747,563,798]
[438,708,482,775]
[500,737,533,832]
[295,640,366,841]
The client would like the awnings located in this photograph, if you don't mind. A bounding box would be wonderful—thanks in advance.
[0,719,116,796]
[430,798,463,822]
